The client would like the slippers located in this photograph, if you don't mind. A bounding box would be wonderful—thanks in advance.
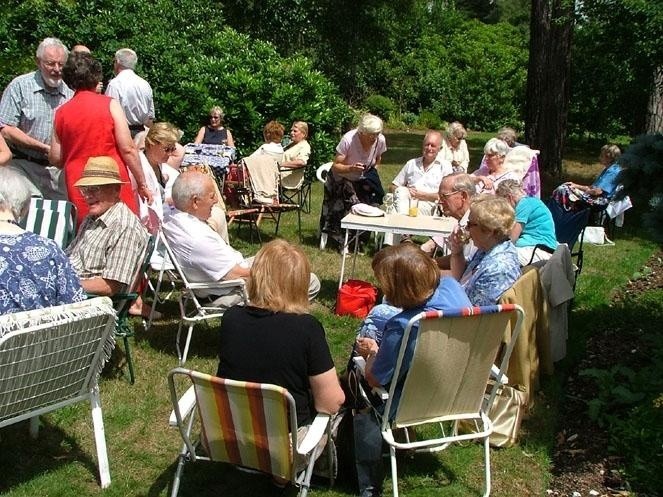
[129,305,164,320]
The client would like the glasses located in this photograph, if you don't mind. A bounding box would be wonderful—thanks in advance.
[438,189,462,200]
[78,185,114,197]
[155,140,177,153]
[466,220,481,228]
[210,116,220,119]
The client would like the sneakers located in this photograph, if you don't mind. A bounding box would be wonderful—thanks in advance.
[354,242,365,256]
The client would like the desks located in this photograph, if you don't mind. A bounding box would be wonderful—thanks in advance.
[337,210,606,291]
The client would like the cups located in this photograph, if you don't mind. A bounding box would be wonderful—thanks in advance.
[409,198,419,217]
[457,225,471,242]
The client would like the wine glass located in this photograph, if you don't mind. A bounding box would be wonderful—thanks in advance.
[359,158,367,180]
[383,195,394,217]
[195,147,202,160]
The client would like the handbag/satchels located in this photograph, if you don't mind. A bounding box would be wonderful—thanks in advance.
[577,226,616,247]
[335,279,379,320]
[339,370,388,496]
[450,378,530,449]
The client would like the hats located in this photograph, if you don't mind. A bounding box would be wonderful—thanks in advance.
[72,156,130,187]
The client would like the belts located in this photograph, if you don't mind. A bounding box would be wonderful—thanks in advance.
[10,147,52,166]
[129,123,145,130]
[194,294,219,305]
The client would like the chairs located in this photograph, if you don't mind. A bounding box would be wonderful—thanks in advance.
[522,206,595,312]
[74,229,154,384]
[16,196,78,254]
[345,303,529,494]
[158,231,251,368]
[180,142,633,245]
[1,295,121,486]
[471,261,550,447]
[162,365,337,496]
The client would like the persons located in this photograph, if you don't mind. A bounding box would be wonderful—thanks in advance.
[1,166,89,315]
[0,38,157,229]
[217,238,475,494]
[64,157,149,313]
[128,105,623,309]
